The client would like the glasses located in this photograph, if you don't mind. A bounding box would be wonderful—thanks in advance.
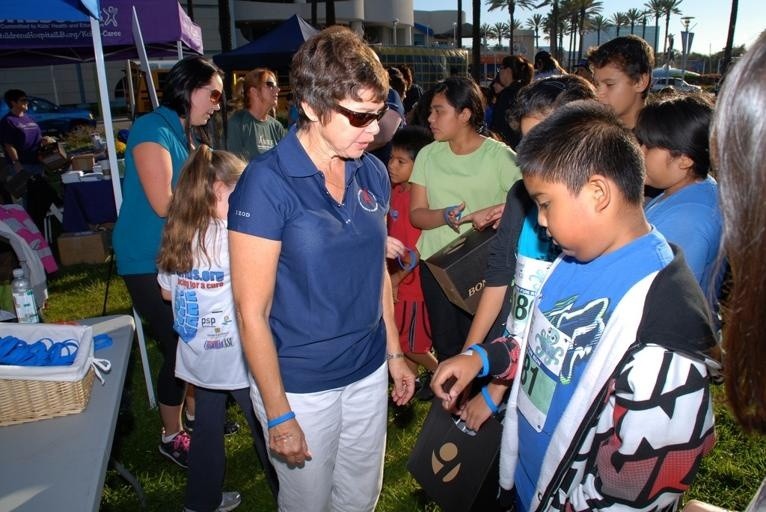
[264,81,274,88]
[496,65,508,73]
[324,97,390,129]
[199,86,225,105]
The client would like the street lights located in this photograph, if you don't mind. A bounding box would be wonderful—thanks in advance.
[393,17,401,44]
[681,15,695,77]
[452,22,458,48]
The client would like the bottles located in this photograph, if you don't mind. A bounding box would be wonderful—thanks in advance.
[11,267,39,324]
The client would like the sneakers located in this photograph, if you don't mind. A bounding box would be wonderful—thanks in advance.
[182,408,241,438]
[158,428,192,470]
[182,490,242,511]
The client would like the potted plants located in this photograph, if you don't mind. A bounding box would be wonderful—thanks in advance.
[65,122,96,174]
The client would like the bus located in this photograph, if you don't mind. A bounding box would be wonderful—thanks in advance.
[219,64,294,130]
[121,57,250,124]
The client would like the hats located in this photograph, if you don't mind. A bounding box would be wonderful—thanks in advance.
[533,51,549,69]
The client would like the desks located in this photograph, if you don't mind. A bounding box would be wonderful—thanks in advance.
[1,312,146,511]
[59,142,133,318]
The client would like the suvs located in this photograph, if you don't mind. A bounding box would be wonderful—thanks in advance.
[651,76,703,96]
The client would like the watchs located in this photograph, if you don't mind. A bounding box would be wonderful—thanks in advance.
[11,159,16,163]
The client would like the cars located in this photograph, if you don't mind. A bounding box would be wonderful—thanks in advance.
[1,95,98,138]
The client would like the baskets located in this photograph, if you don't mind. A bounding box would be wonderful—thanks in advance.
[0,321,95,432]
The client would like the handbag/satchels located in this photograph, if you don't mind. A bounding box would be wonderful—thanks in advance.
[405,396,505,512]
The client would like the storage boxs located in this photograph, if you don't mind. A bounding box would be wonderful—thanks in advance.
[56,229,108,265]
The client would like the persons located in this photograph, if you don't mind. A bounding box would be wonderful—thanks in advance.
[0,89,55,232]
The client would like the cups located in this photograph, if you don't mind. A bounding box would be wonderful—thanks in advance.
[100,161,111,180]
